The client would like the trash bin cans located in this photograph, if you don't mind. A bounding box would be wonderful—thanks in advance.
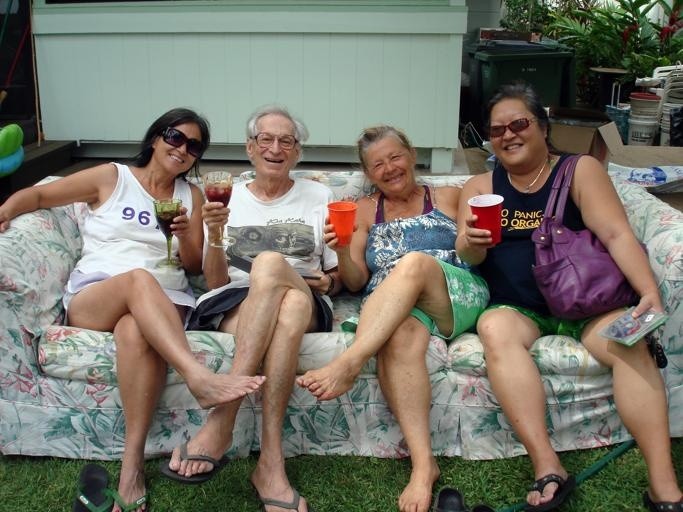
[463,39,575,115]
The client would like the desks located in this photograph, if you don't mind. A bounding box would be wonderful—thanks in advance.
[589,67,637,107]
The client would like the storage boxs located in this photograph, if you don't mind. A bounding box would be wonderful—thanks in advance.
[546,120,625,163]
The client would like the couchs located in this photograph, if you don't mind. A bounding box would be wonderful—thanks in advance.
[0,172,683,464]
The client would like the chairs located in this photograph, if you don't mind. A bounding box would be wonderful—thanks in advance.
[648,65,683,128]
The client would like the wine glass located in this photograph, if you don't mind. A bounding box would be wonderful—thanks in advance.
[153,198,184,270]
[201,171,237,248]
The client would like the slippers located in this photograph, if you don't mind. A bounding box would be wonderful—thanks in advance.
[525,474,577,512]
[73,464,112,512]
[471,502,499,512]
[109,489,150,511]
[155,441,227,484]
[643,489,683,511]
[433,483,470,511]
[248,472,311,512]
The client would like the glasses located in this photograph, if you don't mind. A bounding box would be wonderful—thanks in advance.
[161,126,205,159]
[250,131,299,151]
[487,113,542,139]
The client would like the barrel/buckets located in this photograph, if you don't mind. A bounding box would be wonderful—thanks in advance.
[629,96,660,146]
[660,101,681,147]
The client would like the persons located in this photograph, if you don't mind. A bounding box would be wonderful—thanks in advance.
[454,84,683,512]
[1,107,268,511]
[163,109,339,512]
[294,126,492,511]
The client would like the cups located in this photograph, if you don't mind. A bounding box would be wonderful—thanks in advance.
[326,201,359,246]
[467,193,505,245]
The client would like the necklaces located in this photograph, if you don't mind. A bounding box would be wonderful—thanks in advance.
[507,157,548,198]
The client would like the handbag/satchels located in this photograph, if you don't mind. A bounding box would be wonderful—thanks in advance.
[531,218,648,318]
